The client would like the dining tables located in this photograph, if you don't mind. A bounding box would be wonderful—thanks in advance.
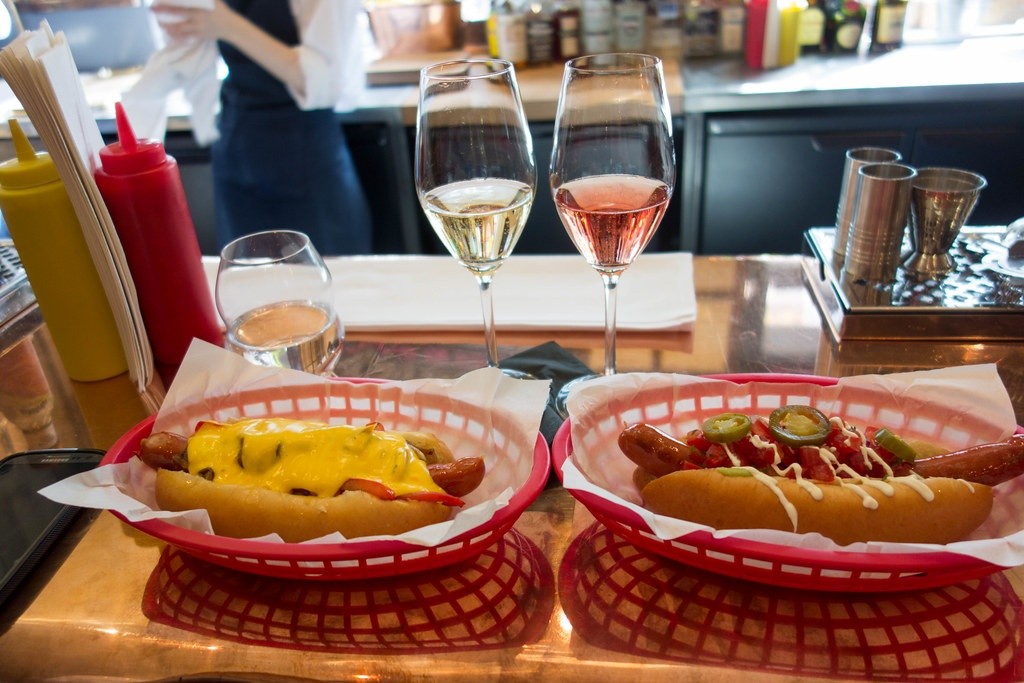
[0,244,1024,683]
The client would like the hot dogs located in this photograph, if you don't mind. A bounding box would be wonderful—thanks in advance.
[138,420,487,544]
[619,405,1024,544]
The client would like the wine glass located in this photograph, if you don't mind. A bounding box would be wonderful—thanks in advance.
[547,51,675,420]
[414,57,538,382]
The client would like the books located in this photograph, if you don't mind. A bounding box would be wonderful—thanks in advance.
[0,17,154,392]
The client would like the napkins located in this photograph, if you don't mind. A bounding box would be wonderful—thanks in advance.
[199,250,699,332]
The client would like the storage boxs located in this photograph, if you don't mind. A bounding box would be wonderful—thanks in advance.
[803,225,1024,343]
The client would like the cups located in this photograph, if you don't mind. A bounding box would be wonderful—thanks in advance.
[213,230,345,378]
[900,167,989,277]
[843,163,919,283]
[830,146,903,265]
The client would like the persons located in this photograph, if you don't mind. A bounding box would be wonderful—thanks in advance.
[151,0,378,255]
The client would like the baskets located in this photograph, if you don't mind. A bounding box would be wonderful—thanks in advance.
[141,528,556,656]
[95,378,551,579]
[551,372,1024,594]
[557,521,1024,683]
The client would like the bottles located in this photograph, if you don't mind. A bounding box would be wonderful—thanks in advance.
[0,115,133,382]
[90,100,226,368]
[484,0,909,71]
